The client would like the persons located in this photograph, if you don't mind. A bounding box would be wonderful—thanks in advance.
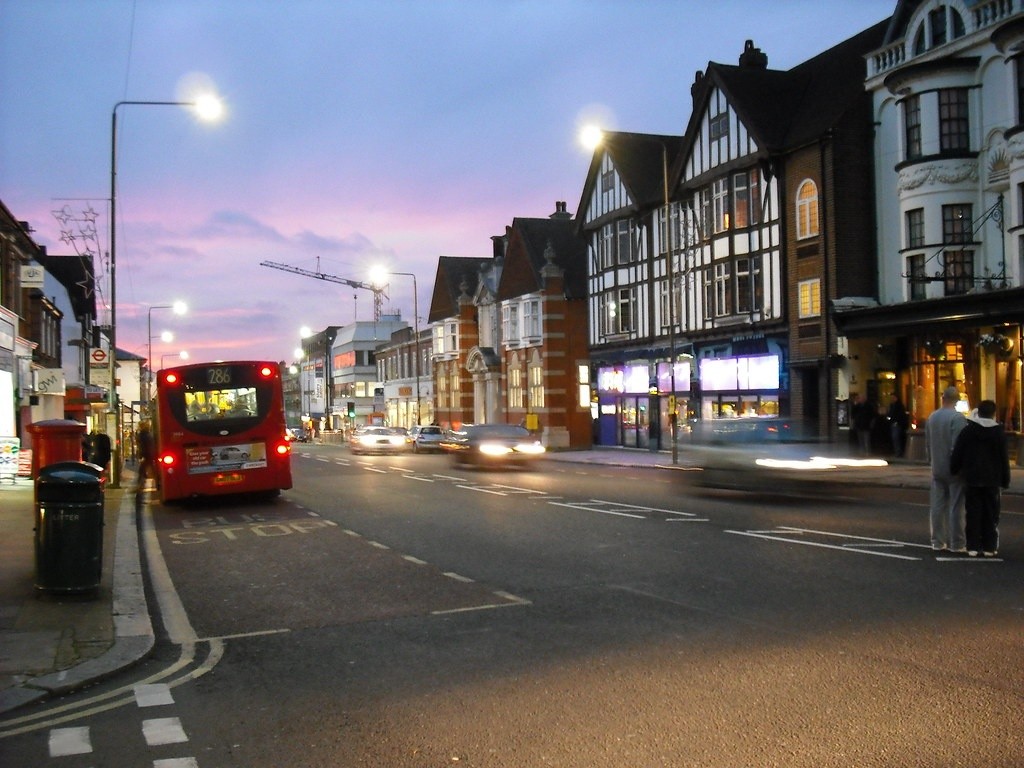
[191,394,250,416]
[81,428,111,468]
[136,420,155,490]
[950,399,1010,556]
[924,386,968,552]
[887,392,907,457]
[851,394,877,457]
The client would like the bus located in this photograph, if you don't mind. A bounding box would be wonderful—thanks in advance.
[145,360,293,508]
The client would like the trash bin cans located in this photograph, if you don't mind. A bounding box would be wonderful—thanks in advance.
[35,472,103,593]
[40,459,106,488]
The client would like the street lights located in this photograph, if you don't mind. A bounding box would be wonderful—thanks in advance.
[149,304,185,402]
[291,349,310,417]
[110,99,220,491]
[373,266,421,426]
[302,327,330,429]
[583,126,681,462]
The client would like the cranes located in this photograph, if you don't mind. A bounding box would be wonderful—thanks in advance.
[260,260,391,325]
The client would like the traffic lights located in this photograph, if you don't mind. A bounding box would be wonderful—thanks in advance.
[348,402,355,417]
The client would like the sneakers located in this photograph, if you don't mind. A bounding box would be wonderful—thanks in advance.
[933,544,947,549]
[983,551,997,557]
[949,547,966,552]
[969,551,978,556]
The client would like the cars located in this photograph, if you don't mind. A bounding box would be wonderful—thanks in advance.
[454,424,541,472]
[681,419,888,498]
[405,425,443,453]
[292,430,309,443]
[350,425,407,457]
